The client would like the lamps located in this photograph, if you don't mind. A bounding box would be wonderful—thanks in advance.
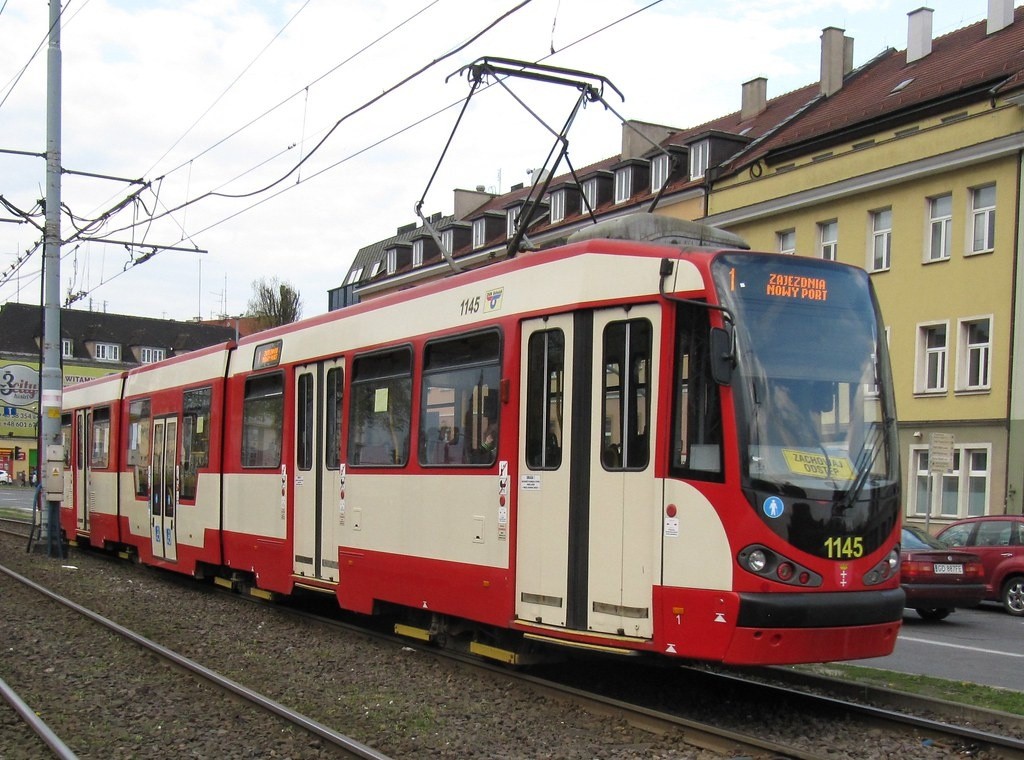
[913,431,922,437]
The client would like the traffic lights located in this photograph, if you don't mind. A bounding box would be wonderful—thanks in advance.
[15,445,22,460]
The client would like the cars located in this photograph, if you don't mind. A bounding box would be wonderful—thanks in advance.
[0,469,14,485]
[898,524,984,624]
[927,514,1024,617]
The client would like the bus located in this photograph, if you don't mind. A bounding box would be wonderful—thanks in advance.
[37,212,906,669]
